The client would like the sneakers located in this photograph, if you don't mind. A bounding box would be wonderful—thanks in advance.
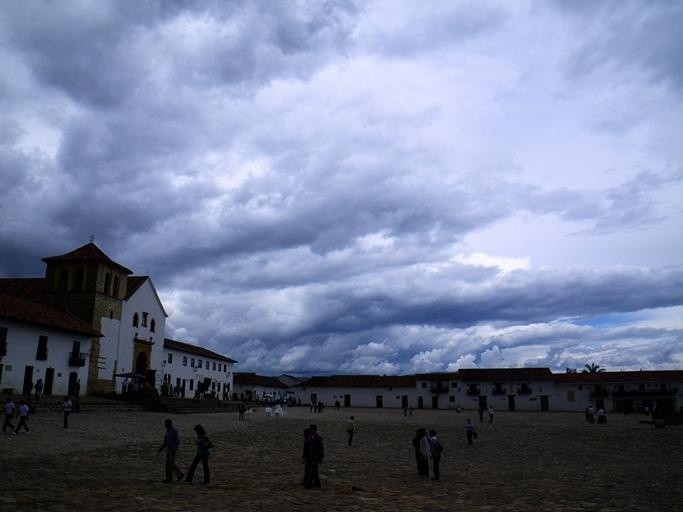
[163,474,192,484]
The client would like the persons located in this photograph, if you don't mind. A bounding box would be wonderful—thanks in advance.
[182,423,214,485]
[25,378,43,399]
[0,395,17,434]
[75,378,80,396]
[345,415,354,446]
[59,395,72,428]
[412,426,442,481]
[10,398,31,434]
[463,418,474,445]
[584,404,604,424]
[488,405,495,424]
[307,423,325,488]
[121,377,340,422]
[301,428,317,489]
[157,418,184,484]
[478,403,484,422]
